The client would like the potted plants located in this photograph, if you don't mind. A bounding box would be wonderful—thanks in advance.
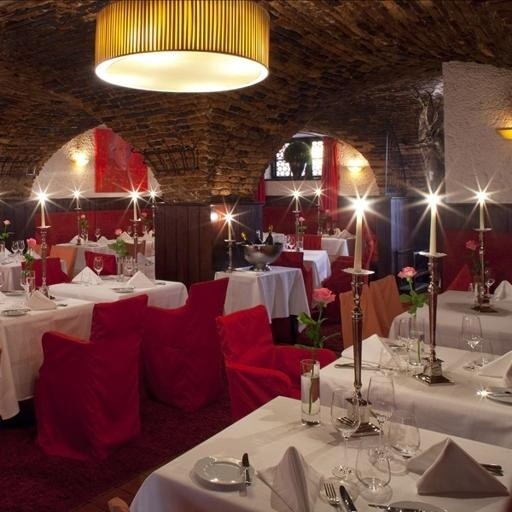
[284,139,312,178]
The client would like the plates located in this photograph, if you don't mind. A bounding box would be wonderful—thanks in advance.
[4,291,24,296]
[384,501,449,512]
[112,287,134,293]
[193,457,255,487]
[484,386,512,405]
[1,309,25,316]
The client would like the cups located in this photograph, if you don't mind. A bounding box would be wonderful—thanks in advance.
[126,226,132,235]
[386,407,422,457]
[379,342,403,378]
[472,337,495,366]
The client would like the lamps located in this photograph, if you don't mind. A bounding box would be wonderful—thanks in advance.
[68,141,88,170]
[94,2,273,93]
[498,127,511,143]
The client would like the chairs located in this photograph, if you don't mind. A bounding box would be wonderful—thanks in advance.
[20,256,69,286]
[370,275,407,331]
[35,294,149,461]
[122,241,146,259]
[216,304,335,418]
[148,276,231,411]
[83,248,119,276]
[269,250,304,268]
[339,286,383,348]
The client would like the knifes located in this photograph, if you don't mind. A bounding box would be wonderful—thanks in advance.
[243,453,251,485]
[335,362,377,370]
[338,484,359,512]
[367,502,420,512]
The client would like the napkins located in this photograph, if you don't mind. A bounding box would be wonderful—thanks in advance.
[3,247,12,260]
[126,271,154,288]
[338,334,404,372]
[72,266,103,285]
[71,231,133,244]
[27,290,57,311]
[493,280,512,300]
[474,349,512,378]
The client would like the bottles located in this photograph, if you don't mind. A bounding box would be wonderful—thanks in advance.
[239,224,275,244]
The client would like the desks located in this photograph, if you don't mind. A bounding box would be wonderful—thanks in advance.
[2,248,64,290]
[257,230,358,258]
[388,289,512,353]
[124,392,512,512]
[216,263,311,322]
[49,271,189,315]
[314,335,512,444]
[1,289,90,417]
[50,235,154,272]
[281,248,333,286]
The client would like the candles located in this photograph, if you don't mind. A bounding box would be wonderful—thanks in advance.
[429,203,437,255]
[75,195,80,209]
[133,194,138,219]
[354,209,364,274]
[151,195,156,207]
[40,201,45,227]
[295,191,298,210]
[228,218,232,241]
[317,192,321,205]
[480,200,484,228]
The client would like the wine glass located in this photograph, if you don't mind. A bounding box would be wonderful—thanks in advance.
[355,440,394,504]
[9,240,25,258]
[278,234,296,252]
[460,316,481,373]
[125,257,134,280]
[485,269,495,297]
[94,227,102,242]
[398,318,416,365]
[367,376,395,458]
[330,389,361,479]
[93,256,104,277]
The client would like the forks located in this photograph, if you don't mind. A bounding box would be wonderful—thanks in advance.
[324,481,344,512]
